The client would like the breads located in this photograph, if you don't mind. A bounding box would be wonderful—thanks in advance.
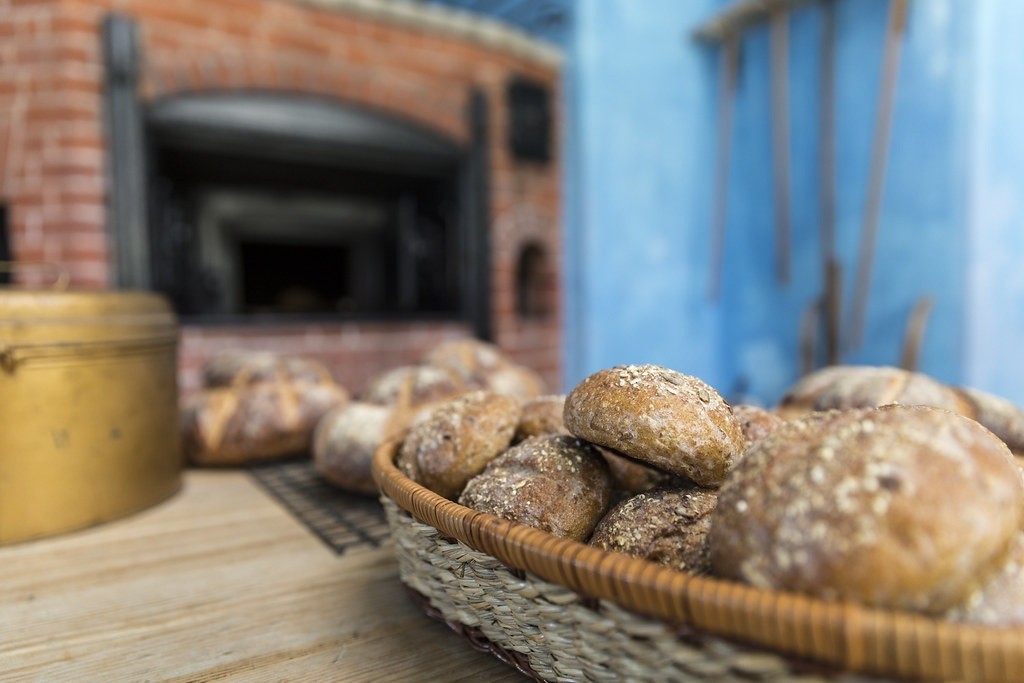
[183,338,1024,633]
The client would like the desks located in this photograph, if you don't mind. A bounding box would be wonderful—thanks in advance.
[1,452,536,683]
